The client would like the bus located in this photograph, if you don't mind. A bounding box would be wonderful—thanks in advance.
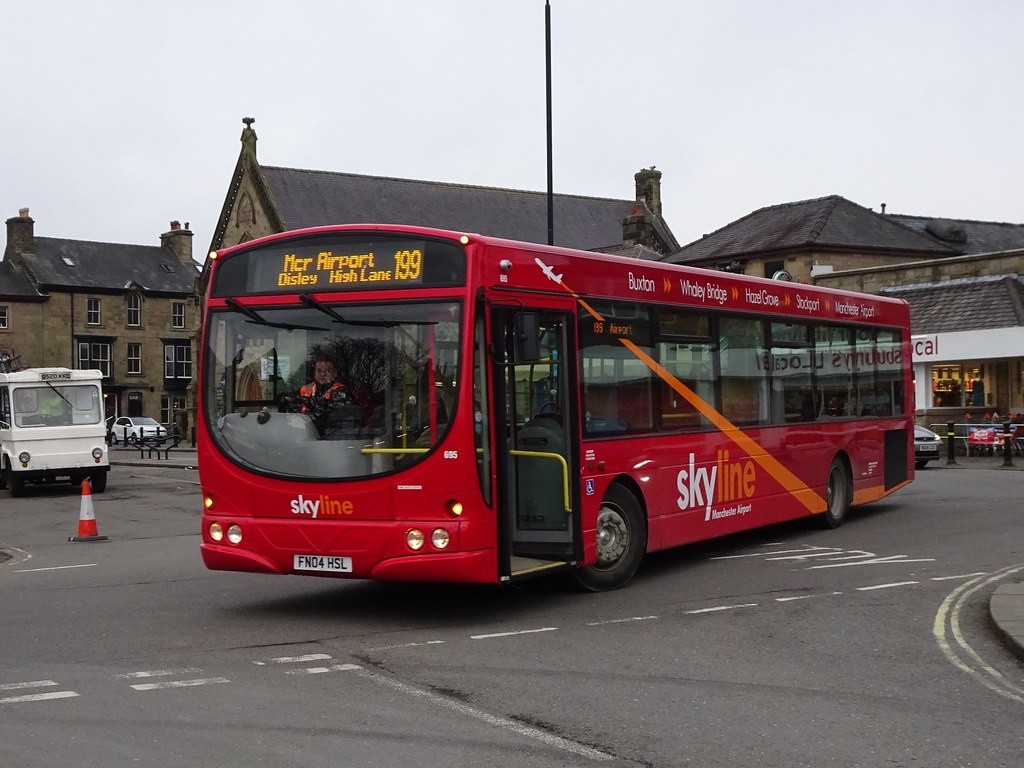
[198,223,917,594]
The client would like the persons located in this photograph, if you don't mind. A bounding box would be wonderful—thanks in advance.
[299,355,346,414]
[946,385,961,406]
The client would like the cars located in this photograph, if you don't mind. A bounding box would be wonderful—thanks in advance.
[913,424,944,471]
[111,416,167,445]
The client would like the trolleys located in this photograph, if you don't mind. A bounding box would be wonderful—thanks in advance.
[962,411,1024,458]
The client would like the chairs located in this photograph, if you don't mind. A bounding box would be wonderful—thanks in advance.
[785,385,890,421]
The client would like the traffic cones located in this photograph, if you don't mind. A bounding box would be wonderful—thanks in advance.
[68,477,108,541]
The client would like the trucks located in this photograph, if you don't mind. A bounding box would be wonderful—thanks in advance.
[0,367,111,498]
